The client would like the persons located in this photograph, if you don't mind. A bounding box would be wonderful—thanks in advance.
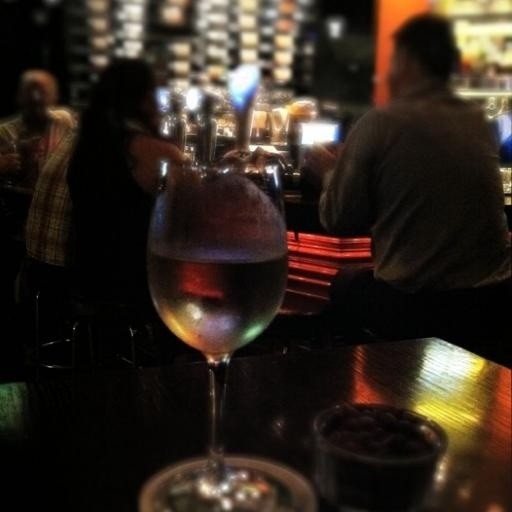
[301,10,511,343]
[26,58,192,292]
[1,72,74,228]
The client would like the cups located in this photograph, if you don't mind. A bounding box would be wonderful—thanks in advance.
[317,401,451,511]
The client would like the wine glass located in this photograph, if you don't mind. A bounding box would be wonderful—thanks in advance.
[137,154,317,512]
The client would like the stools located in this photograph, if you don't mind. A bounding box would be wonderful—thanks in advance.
[13,241,178,430]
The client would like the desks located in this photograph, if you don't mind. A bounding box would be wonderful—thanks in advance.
[0,333,512,511]
